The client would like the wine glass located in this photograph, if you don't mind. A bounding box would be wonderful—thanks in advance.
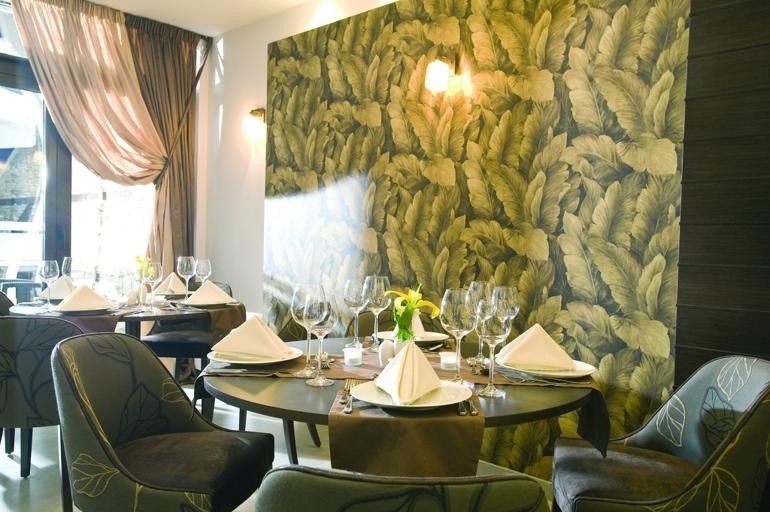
[175,256,211,308]
[439,280,516,398]
[346,277,367,352]
[307,293,339,387]
[41,255,85,312]
[146,262,163,314]
[362,272,393,347]
[296,282,328,378]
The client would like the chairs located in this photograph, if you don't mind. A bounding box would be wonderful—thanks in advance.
[0,280,48,306]
[0,315,85,478]
[252,465,554,511]
[0,291,16,318]
[546,354,769,511]
[45,331,276,511]
[189,298,330,461]
[139,282,234,388]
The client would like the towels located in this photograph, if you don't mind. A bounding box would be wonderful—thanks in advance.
[55,282,115,312]
[388,304,426,336]
[153,271,192,295]
[207,311,298,358]
[371,340,444,407]
[183,278,236,306]
[37,275,76,301]
[496,324,580,375]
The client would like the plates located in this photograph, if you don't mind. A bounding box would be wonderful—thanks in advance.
[349,380,471,412]
[206,347,302,367]
[494,355,597,380]
[34,292,237,312]
[373,330,448,346]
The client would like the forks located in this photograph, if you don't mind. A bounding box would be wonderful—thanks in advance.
[337,379,364,415]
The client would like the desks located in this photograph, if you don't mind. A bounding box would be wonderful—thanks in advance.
[203,335,605,441]
[8,297,208,342]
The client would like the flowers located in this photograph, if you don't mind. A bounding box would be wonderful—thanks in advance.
[129,257,159,283]
[380,281,441,320]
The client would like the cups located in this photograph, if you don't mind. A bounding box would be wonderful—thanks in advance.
[342,349,363,366]
[439,352,457,371]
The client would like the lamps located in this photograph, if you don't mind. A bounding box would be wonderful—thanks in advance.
[241,108,267,138]
[424,41,462,95]
